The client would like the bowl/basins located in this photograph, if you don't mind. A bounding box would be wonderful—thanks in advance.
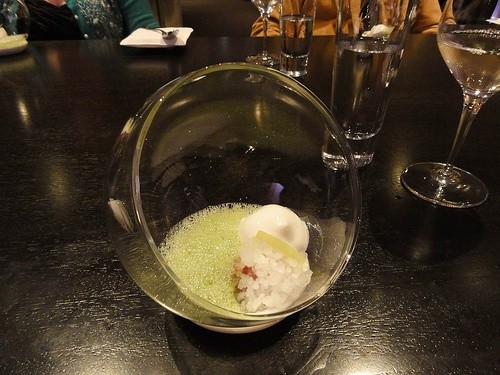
[100,59,360,329]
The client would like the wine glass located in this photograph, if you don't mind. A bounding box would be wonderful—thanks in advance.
[399,0,500,209]
[245,0,280,66]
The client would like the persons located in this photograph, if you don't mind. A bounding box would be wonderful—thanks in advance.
[0,0,500,42]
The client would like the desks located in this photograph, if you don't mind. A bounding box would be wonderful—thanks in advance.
[0,32,500,375]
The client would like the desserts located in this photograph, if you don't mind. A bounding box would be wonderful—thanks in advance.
[236,203,313,315]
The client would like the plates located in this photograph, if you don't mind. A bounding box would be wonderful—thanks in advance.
[0,42,28,54]
[137,274,334,333]
[119,26,194,48]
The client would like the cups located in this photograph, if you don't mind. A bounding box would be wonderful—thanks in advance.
[279,0,317,77]
[321,0,423,172]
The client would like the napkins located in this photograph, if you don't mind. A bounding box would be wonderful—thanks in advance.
[120,27,193,47]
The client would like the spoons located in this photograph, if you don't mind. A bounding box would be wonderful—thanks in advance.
[141,23,180,39]
